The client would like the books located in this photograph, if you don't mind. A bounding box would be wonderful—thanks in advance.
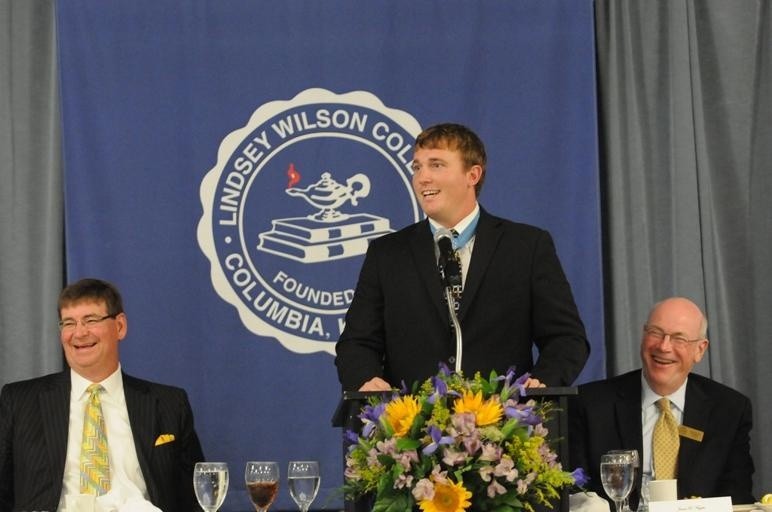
[270,211,390,245]
[256,229,398,266]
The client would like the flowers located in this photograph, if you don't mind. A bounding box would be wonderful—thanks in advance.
[320,361,591,512]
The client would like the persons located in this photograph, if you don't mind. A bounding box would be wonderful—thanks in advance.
[569,296,755,512]
[333,123,593,395]
[0,278,206,512]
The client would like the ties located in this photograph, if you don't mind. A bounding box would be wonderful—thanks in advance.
[439,230,463,318]
[652,398,680,480]
[80,384,110,497]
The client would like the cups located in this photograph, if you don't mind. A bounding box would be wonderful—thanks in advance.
[644,479,680,501]
[193,459,323,512]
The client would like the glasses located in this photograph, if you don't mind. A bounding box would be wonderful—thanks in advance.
[643,324,701,346]
[58,314,113,329]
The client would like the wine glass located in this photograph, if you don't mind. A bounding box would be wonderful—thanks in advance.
[598,449,638,512]
